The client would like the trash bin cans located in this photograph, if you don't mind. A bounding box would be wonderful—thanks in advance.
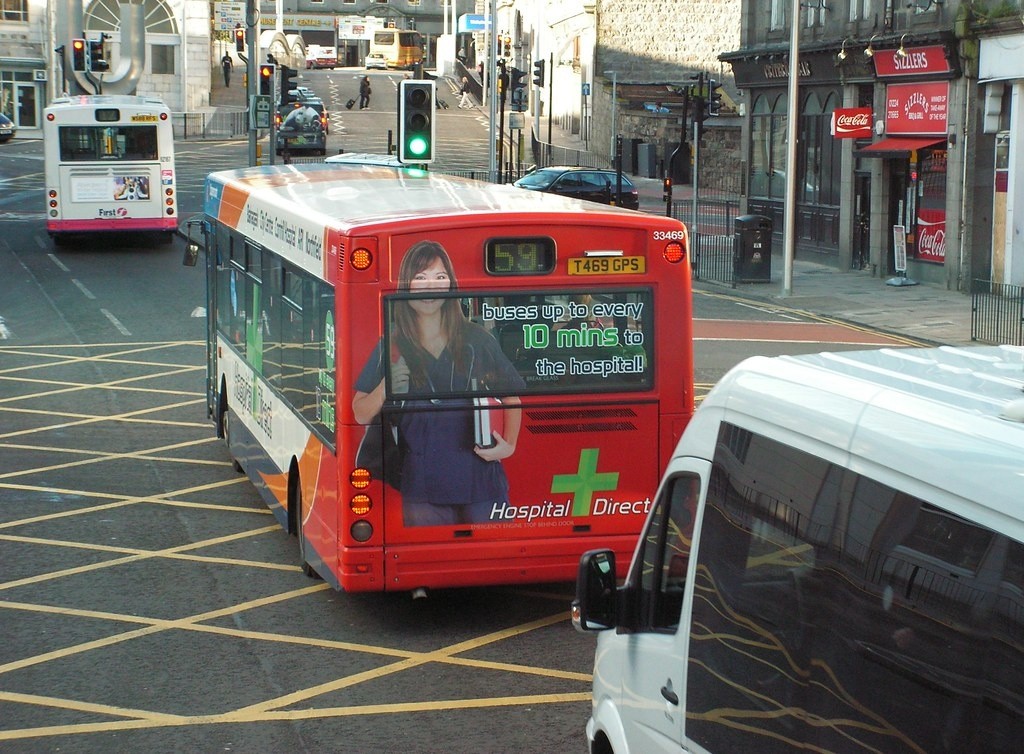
[734,214,771,285]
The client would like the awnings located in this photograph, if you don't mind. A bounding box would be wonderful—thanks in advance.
[852,136,948,159]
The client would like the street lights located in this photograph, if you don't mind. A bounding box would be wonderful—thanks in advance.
[602,70,616,169]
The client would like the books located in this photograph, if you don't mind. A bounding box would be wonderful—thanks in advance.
[470,377,505,449]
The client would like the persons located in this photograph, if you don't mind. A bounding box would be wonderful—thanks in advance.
[220,51,234,88]
[403,73,411,79]
[351,239,528,526]
[359,76,372,110]
[559,293,604,331]
[496,293,560,331]
[479,67,484,85]
[458,77,474,109]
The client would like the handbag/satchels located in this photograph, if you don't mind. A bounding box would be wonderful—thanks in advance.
[354,337,409,490]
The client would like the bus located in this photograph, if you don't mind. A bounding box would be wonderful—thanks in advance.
[373,29,426,71]
[305,45,338,70]
[568,343,1024,754]
[41,95,180,237]
[179,159,698,597]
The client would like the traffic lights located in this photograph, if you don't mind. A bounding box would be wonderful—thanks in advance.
[87,39,110,73]
[279,64,299,107]
[511,67,528,103]
[388,22,396,29]
[71,38,88,74]
[257,64,276,97]
[533,59,545,87]
[664,178,672,192]
[698,96,710,140]
[707,78,725,117]
[233,28,245,52]
[497,35,502,56]
[398,78,438,166]
[504,36,511,57]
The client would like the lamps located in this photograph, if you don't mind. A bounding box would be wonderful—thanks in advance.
[838,37,858,60]
[896,33,919,58]
[863,34,887,57]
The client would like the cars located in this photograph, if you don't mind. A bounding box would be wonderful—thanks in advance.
[0,111,17,144]
[364,51,388,70]
[276,85,331,156]
[510,163,642,212]
